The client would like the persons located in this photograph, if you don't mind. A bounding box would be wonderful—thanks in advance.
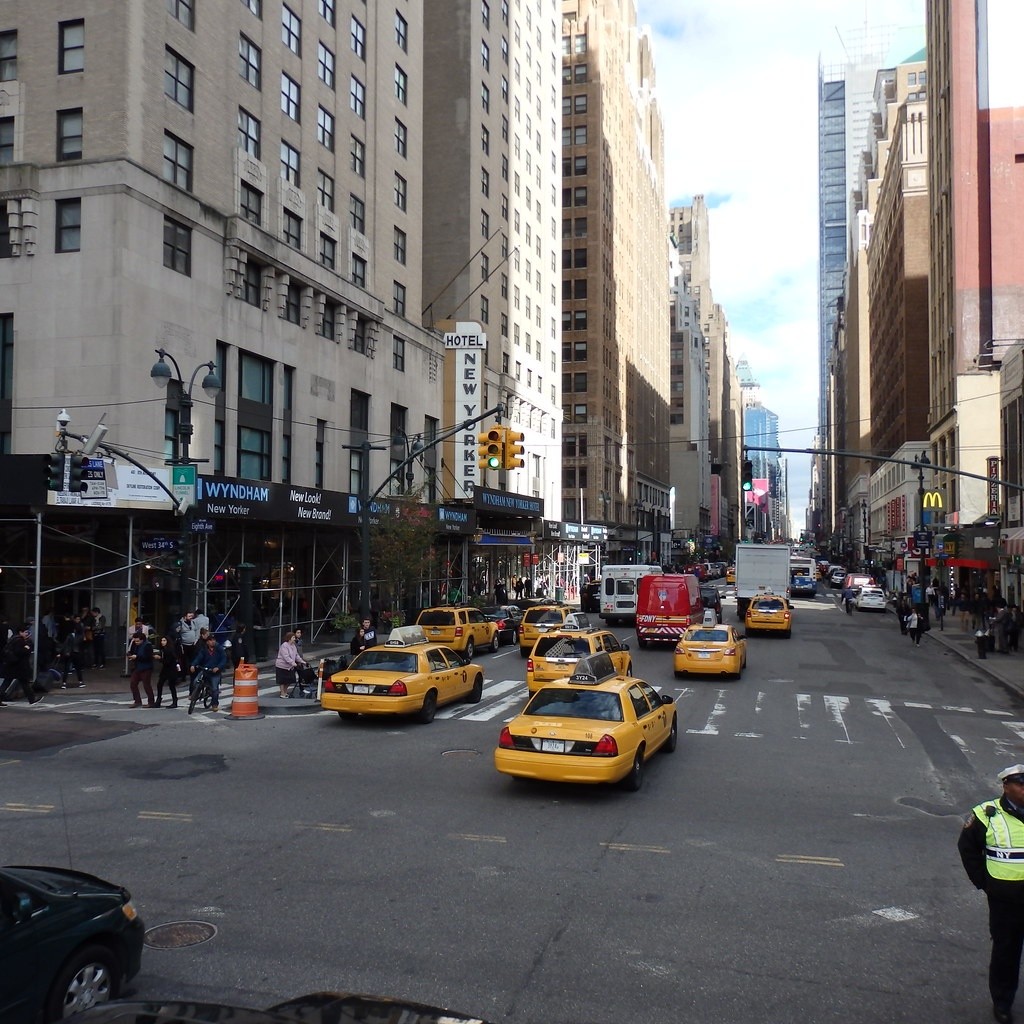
[153,636,178,708]
[350,628,366,662]
[958,763,1024,1024]
[0,606,108,708]
[128,594,353,684]
[291,628,312,694]
[449,572,591,606]
[897,573,1024,654]
[188,627,227,712]
[361,617,376,664]
[129,633,155,708]
[842,588,856,615]
[275,632,306,698]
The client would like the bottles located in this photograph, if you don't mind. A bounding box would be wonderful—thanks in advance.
[177,664,181,672]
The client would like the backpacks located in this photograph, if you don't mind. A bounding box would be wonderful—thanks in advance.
[1,636,23,667]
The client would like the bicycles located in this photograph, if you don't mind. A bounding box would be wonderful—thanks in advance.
[189,665,223,714]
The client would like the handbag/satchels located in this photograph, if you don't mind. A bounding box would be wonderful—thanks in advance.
[61,652,71,660]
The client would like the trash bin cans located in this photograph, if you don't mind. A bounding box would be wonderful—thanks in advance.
[555,586,564,602]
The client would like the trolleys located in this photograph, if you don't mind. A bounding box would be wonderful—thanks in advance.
[290,663,319,699]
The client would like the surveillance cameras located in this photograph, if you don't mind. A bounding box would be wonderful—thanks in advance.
[57,412,71,425]
[82,424,108,456]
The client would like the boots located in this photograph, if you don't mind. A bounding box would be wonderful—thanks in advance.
[169,697,178,707]
[151,698,162,708]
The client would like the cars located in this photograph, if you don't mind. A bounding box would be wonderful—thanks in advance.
[479,606,526,645]
[690,562,736,585]
[817,561,886,610]
[0,866,147,1023]
[514,599,562,612]
[321,626,485,725]
[674,609,747,680]
[495,652,678,793]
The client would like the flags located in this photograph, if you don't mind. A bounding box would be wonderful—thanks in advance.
[748,479,769,513]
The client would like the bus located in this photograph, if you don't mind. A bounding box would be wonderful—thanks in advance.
[790,556,817,599]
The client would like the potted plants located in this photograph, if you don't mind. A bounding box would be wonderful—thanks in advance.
[334,614,358,642]
[382,612,402,633]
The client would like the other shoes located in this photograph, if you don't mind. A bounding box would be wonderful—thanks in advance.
[213,705,218,712]
[994,1005,1012,1023]
[91,664,98,670]
[79,684,86,687]
[129,702,142,707]
[99,664,106,670]
[142,704,151,708]
[60,683,66,688]
[280,694,289,699]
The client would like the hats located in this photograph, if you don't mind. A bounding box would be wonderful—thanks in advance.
[999,765,1024,783]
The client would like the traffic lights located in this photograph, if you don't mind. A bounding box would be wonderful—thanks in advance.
[478,425,524,471]
[800,533,803,541]
[740,459,752,492]
[46,453,89,494]
[175,532,193,570]
[689,534,693,542]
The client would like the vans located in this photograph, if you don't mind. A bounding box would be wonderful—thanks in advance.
[699,586,727,624]
[843,572,874,596]
[635,573,710,650]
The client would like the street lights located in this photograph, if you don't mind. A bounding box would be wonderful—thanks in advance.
[392,426,424,624]
[911,452,932,632]
[152,348,222,614]
[861,499,868,573]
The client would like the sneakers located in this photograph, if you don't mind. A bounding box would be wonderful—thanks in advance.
[30,696,44,705]
[0,703,8,708]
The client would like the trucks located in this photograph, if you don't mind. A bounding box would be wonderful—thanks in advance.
[735,544,792,623]
[599,564,665,625]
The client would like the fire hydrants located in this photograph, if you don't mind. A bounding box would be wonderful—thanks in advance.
[975,631,988,659]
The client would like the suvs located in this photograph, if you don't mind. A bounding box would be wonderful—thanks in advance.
[519,599,581,657]
[745,585,795,639]
[582,581,601,612]
[526,612,632,700]
[416,607,499,660]
